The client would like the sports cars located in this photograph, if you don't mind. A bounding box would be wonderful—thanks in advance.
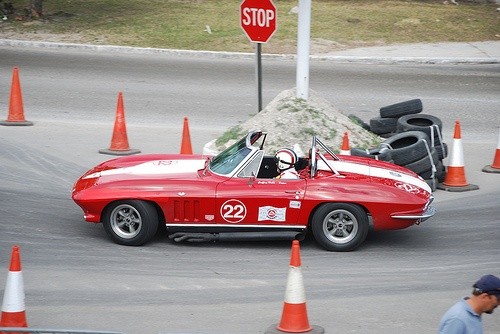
[69,129,438,252]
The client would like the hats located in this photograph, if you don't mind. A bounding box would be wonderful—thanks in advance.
[473,274,500,292]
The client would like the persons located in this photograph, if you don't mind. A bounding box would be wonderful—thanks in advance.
[436,275,500,334]
[273,148,300,180]
[2,2,41,18]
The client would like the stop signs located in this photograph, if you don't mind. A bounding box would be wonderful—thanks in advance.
[239,0,279,44]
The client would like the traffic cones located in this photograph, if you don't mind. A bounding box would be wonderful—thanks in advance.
[340,133,353,157]
[97,92,142,156]
[178,117,193,155]
[263,240,325,334]
[1,245,33,334]
[480,128,500,173]
[436,119,480,192]
[0,66,34,127]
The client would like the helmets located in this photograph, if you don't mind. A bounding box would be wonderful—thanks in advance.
[273,147,298,170]
